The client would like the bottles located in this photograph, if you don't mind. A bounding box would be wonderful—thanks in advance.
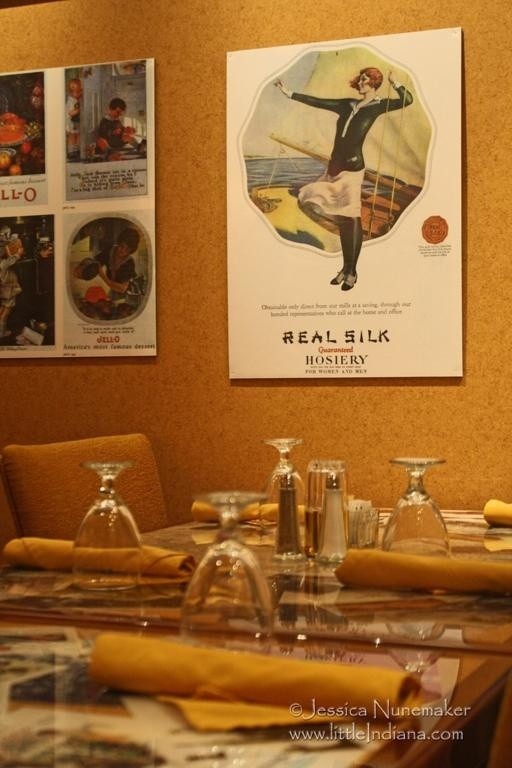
[316,470,348,565]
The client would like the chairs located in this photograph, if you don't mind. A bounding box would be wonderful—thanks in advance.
[0,433,167,540]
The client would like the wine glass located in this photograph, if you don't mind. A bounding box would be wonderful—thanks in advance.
[177,491,274,645]
[272,567,303,656]
[258,436,309,566]
[72,587,148,665]
[70,460,145,593]
[382,455,454,557]
[378,613,452,711]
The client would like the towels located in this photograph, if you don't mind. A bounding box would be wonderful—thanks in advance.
[85,630,426,737]
[334,547,511,604]
[3,536,198,591]
[484,524,512,553]
[190,500,307,528]
[191,526,307,545]
[483,499,511,528]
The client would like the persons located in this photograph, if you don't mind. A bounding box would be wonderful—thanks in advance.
[73,229,141,306]
[98,97,147,153]
[65,79,82,157]
[0,239,25,338]
[272,65,413,292]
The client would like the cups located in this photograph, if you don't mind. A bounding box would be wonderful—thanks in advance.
[346,509,378,550]
[306,457,349,557]
